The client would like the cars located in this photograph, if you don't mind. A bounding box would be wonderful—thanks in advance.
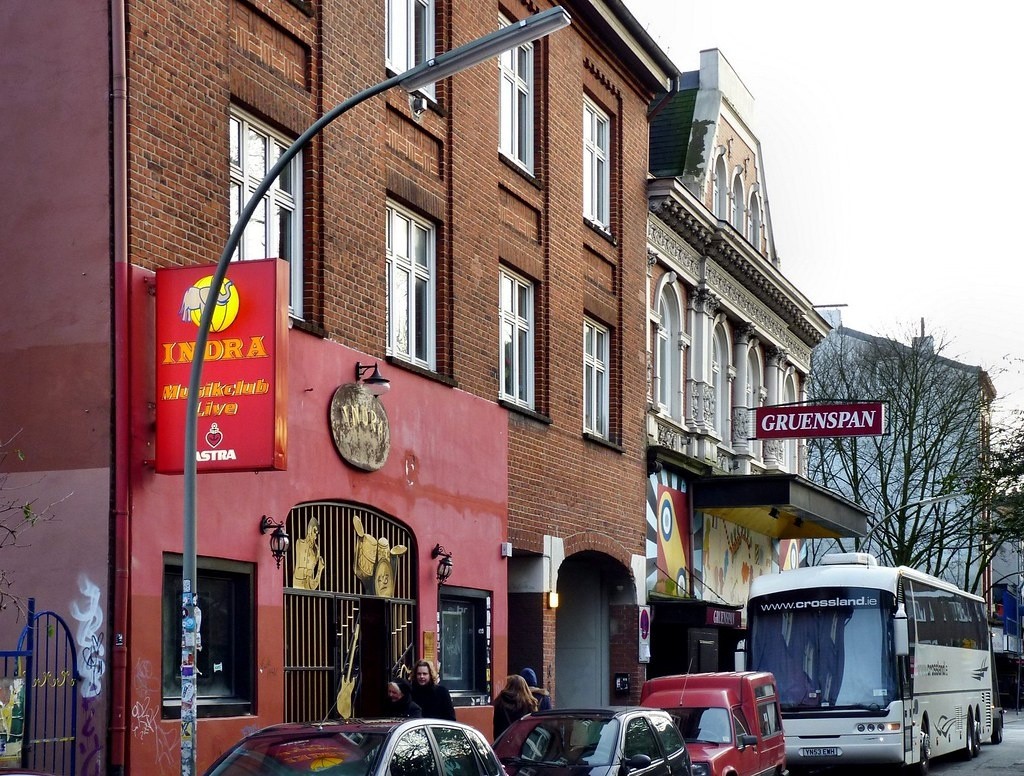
[483,703,694,776]
[202,713,509,776]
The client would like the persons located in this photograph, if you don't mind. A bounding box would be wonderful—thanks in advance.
[411,659,456,721]
[387,677,423,718]
[493,675,540,742]
[521,668,554,711]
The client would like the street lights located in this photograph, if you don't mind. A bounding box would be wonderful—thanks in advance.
[179,6,573,776]
[857,491,959,554]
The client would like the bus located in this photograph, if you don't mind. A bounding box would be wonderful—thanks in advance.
[734,550,994,776]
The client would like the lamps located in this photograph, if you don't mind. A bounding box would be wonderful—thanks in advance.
[432,543,453,587]
[356,361,391,399]
[260,514,290,570]
[768,507,781,520]
[546,592,559,610]
[792,517,804,528]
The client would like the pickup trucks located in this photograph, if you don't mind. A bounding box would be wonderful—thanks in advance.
[631,670,789,776]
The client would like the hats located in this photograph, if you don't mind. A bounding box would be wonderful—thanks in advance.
[392,677,412,696]
[520,668,537,686]
